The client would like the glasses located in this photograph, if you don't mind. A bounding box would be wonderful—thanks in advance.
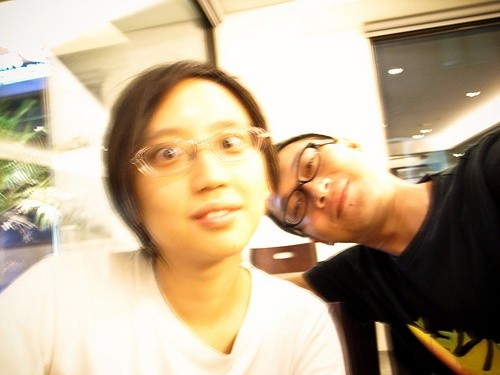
[282,141,333,231]
[128,127,270,177]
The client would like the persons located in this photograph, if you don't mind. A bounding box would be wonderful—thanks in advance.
[262,133,500,375]
[1,60,351,375]
